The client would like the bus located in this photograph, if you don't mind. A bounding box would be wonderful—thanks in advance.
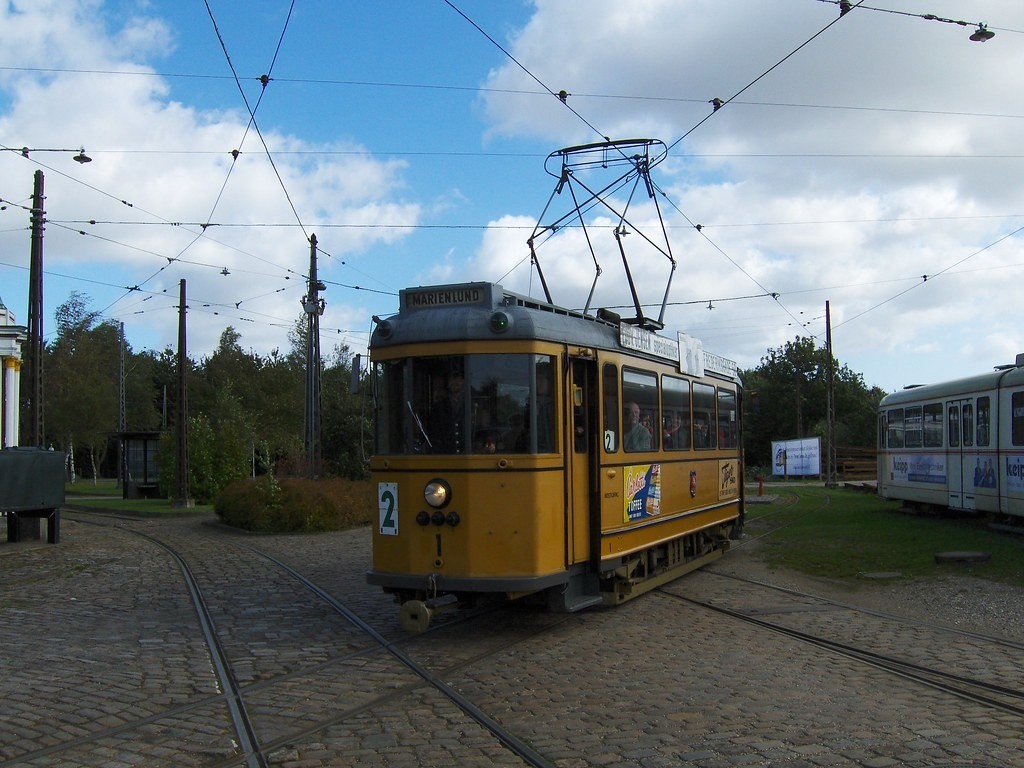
[366,281,747,633]
[877,353,1024,517]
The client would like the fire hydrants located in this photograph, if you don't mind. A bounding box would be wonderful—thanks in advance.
[757,473,766,496]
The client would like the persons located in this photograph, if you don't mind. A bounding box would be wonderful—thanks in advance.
[694,420,710,448]
[412,362,556,455]
[718,416,734,449]
[663,413,691,448]
[623,400,655,450]
